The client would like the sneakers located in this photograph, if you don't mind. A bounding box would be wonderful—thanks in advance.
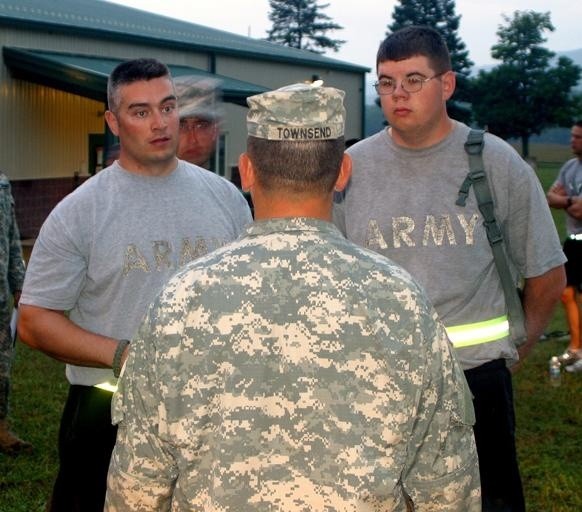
[559,345,582,373]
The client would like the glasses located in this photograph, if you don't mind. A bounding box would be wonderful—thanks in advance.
[372,71,447,93]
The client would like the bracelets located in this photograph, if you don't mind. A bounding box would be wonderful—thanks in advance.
[112,339,130,378]
[568,196,573,206]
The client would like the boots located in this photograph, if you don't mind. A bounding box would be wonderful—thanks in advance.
[1,420,33,454]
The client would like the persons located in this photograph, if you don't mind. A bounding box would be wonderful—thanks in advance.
[17,60,254,512]
[102,84,482,512]
[331,26,568,512]
[171,76,224,171]
[547,120,582,373]
[0,167,37,456]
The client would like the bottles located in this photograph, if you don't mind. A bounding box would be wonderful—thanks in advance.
[550,356,561,387]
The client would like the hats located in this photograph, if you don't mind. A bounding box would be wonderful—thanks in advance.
[173,75,218,122]
[246,79,347,142]
